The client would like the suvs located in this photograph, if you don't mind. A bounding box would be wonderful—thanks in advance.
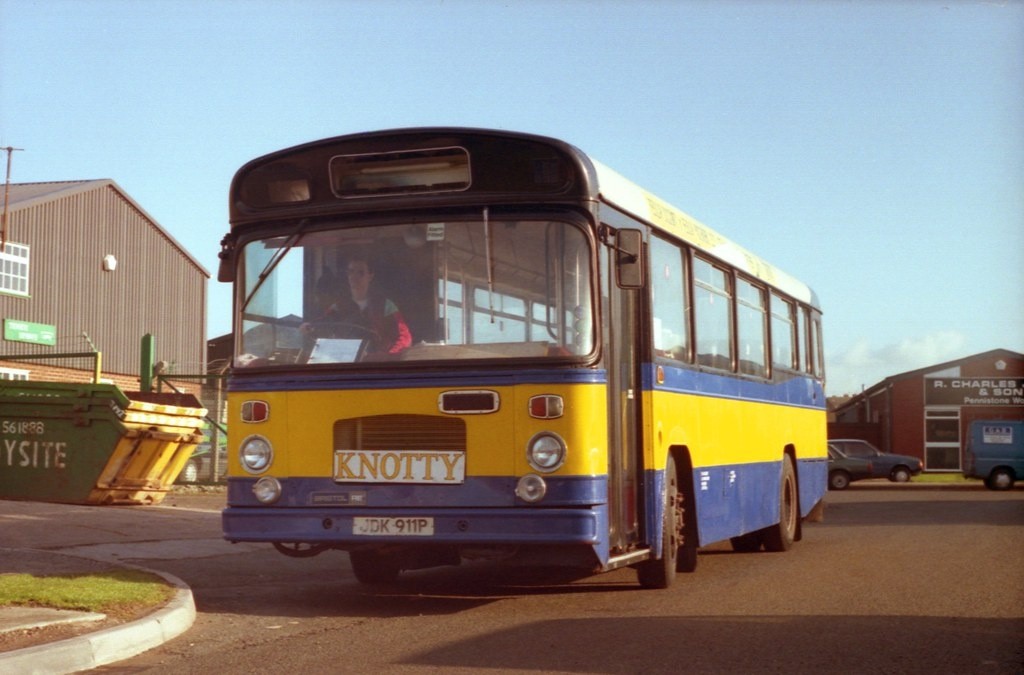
[828,440,923,482]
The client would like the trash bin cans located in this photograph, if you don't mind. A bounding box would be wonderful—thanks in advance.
[0,374,211,508]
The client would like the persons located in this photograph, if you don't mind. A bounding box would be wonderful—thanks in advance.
[299,257,412,358]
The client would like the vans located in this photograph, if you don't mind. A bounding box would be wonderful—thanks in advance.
[965,421,1024,490]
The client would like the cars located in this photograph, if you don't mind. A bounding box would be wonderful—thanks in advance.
[827,444,873,489]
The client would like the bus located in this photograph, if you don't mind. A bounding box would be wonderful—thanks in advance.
[218,127,827,587]
